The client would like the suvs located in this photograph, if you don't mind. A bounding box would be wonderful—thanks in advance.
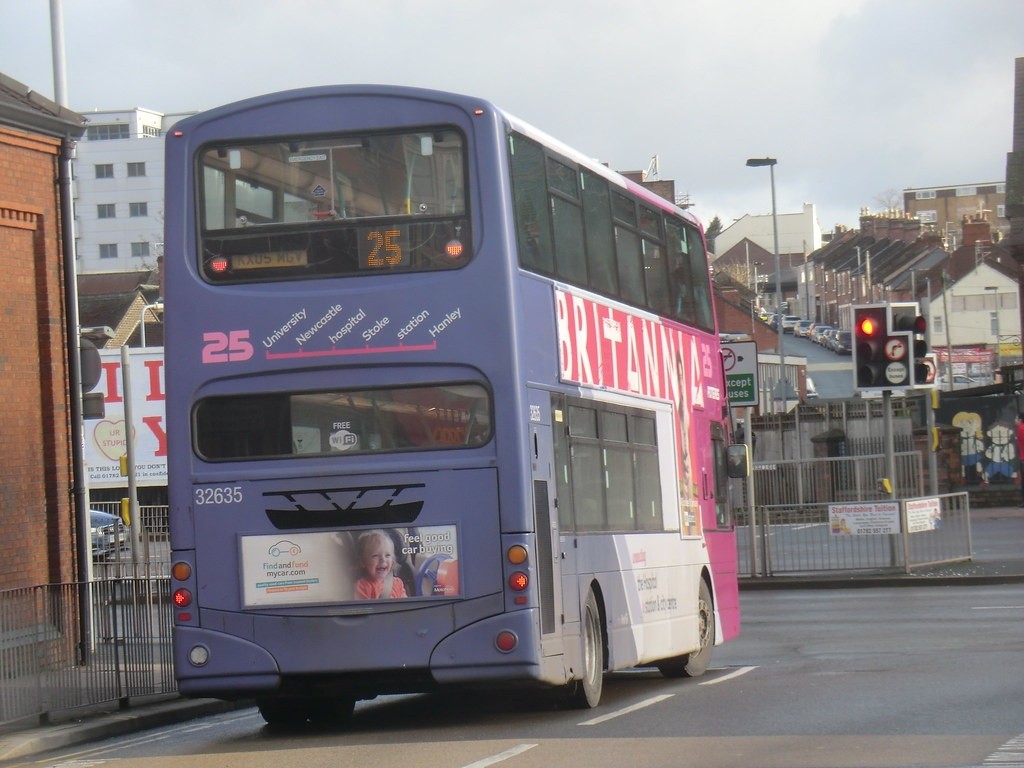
[940,375,977,391]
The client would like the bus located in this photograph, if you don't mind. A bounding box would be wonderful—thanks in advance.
[158,84,737,729]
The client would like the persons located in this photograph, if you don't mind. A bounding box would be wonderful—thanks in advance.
[353,528,410,604]
[1014,412,1024,508]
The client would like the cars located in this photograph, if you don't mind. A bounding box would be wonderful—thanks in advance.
[90,509,128,562]
[794,320,813,337]
[806,323,853,355]
[760,312,801,335]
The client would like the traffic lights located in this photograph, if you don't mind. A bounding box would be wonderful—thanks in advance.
[847,304,892,392]
[890,303,933,388]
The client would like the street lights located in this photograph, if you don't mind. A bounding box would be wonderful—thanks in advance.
[984,285,1003,369]
[140,302,164,349]
[747,156,787,417]
